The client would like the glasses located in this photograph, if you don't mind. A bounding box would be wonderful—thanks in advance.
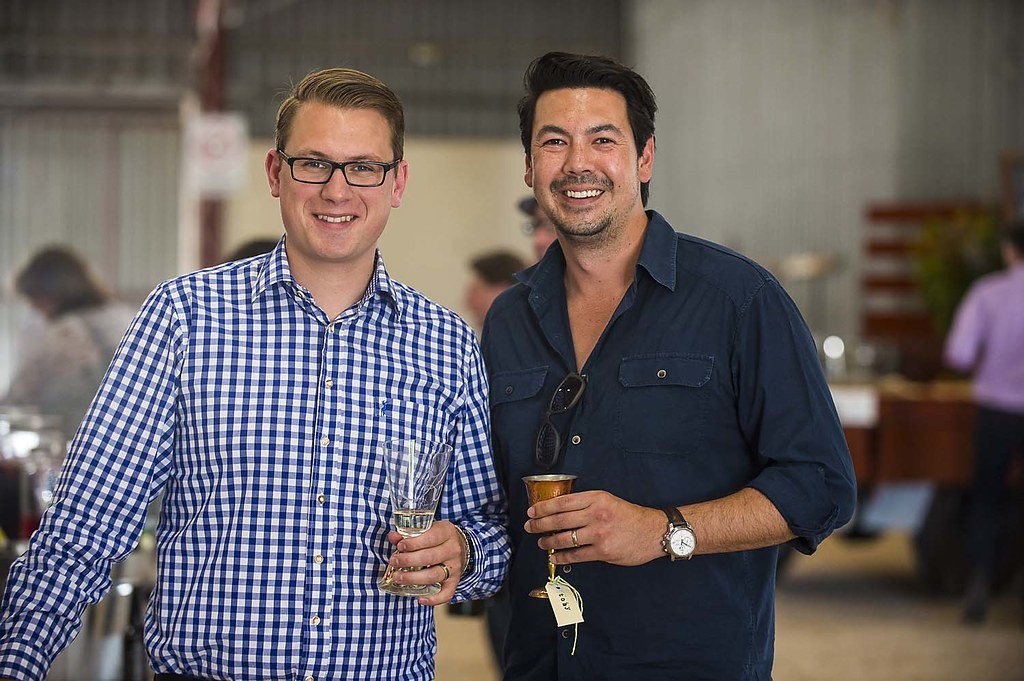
[536,372,590,467]
[277,149,401,187]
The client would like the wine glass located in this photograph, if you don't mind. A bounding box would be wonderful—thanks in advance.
[377,438,453,597]
[524,474,579,599]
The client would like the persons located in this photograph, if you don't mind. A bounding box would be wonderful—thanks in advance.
[0,58,511,681]
[480,50,857,681]
[1,242,140,473]
[942,218,1024,625]
[461,198,557,352]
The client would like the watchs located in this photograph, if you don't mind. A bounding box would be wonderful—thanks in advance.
[658,505,699,562]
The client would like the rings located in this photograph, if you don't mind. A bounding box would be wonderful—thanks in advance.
[570,530,583,548]
[440,561,451,584]
